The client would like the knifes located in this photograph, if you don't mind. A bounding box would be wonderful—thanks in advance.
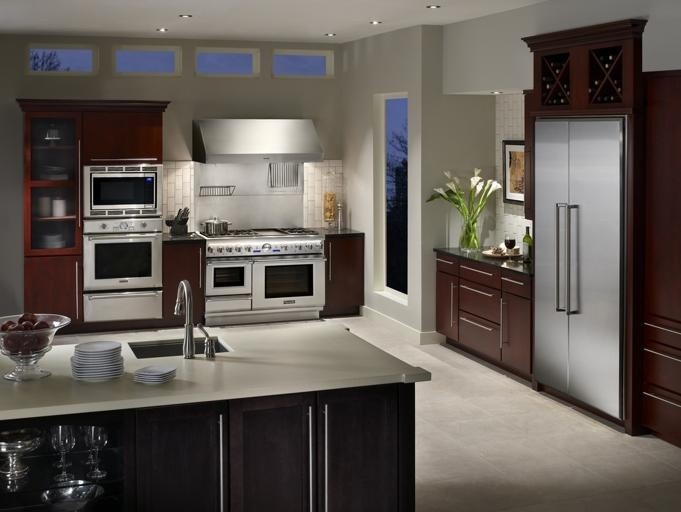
[176,205,190,224]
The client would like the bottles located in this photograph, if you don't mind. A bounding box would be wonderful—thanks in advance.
[335,199,345,229]
[523,226,533,263]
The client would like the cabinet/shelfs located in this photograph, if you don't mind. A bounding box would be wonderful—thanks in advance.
[77,104,166,162]
[162,243,209,320]
[21,104,80,333]
[0,399,224,510]
[436,247,458,345]
[460,256,500,361]
[322,229,365,318]
[499,268,531,380]
[225,377,415,511]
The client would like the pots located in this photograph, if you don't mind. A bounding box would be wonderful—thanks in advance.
[201,218,232,234]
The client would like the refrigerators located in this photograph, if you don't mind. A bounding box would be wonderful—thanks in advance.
[531,117,625,426]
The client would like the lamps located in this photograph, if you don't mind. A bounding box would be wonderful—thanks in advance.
[324,192,336,230]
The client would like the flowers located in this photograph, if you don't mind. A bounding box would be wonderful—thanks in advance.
[432,168,506,245]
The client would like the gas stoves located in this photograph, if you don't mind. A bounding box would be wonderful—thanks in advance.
[198,228,321,238]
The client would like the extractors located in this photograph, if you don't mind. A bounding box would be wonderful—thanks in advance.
[192,119,326,163]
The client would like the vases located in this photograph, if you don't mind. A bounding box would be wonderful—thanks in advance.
[459,215,482,252]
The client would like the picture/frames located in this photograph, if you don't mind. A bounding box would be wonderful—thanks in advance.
[502,139,527,204]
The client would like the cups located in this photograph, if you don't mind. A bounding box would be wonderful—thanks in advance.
[52,200,66,216]
[37,196,51,217]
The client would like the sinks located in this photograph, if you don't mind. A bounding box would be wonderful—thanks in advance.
[126,335,230,360]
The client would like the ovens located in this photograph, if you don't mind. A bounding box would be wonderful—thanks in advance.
[82,234,163,291]
[206,259,252,296]
[252,258,326,311]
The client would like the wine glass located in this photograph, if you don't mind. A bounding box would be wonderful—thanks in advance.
[80,424,108,478]
[505,233,516,263]
[165,215,176,237]
[48,425,76,481]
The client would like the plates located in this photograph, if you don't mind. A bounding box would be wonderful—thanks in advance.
[133,366,175,383]
[39,234,65,248]
[482,250,523,257]
[70,342,124,383]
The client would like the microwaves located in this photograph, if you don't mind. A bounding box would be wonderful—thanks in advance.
[83,164,164,218]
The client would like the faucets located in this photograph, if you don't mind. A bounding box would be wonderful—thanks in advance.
[173,279,197,361]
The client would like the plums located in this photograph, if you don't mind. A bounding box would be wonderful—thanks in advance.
[2,313,49,356]
